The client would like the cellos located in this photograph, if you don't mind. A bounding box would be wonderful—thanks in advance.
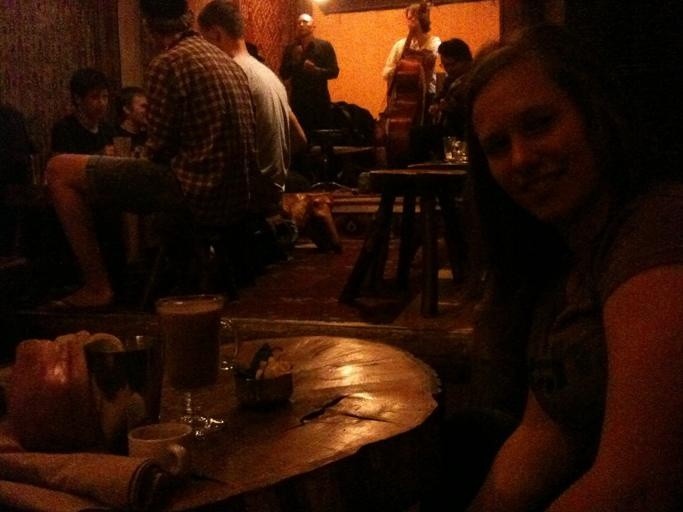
[373,1,435,169]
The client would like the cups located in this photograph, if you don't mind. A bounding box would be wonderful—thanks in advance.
[127,422,193,481]
[82,333,164,444]
[229,369,292,404]
[443,134,469,163]
[112,137,130,154]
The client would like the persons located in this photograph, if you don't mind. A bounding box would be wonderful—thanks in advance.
[246,41,307,146]
[446,18,682,512]
[43,0,257,306]
[278,12,339,128]
[112,84,149,161]
[429,37,472,145]
[195,3,288,217]
[48,68,117,158]
[379,1,441,168]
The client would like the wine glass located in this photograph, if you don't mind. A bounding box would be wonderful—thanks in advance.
[155,294,229,440]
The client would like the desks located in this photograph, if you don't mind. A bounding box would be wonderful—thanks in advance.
[311,118,487,323]
[147,331,445,512]
